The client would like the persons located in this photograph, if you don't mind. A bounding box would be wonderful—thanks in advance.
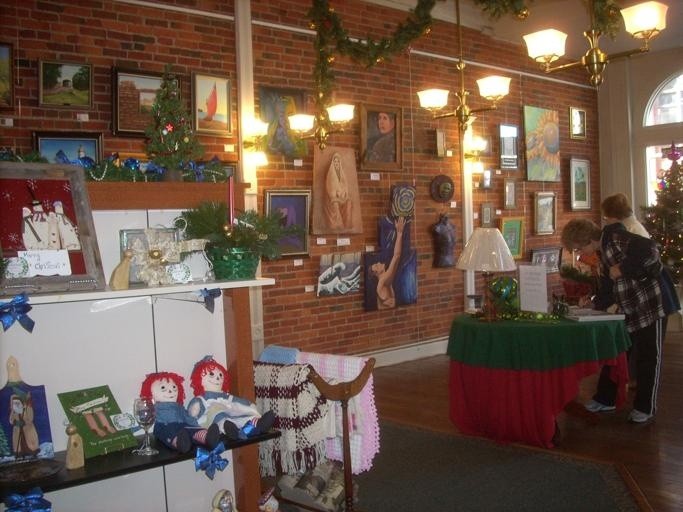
[428,213,457,268]
[8,391,41,458]
[324,153,352,230]
[599,191,650,398]
[561,219,665,421]
[367,217,406,310]
[368,112,395,162]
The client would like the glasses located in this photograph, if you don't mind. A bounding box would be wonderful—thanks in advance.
[573,241,591,254]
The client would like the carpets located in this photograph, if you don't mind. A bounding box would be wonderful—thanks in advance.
[354,421,642,512]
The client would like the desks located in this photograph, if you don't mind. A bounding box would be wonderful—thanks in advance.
[451,310,628,442]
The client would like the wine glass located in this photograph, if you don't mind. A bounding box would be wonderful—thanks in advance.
[130,399,159,455]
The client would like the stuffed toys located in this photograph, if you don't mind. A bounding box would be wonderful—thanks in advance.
[139,371,219,453]
[187,355,278,444]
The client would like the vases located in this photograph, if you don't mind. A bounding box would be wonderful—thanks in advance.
[111,65,235,141]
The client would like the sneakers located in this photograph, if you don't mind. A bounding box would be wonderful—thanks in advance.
[582,399,616,413]
[628,409,655,423]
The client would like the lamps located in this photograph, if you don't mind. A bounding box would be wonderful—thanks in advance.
[415,0,669,135]
[456,227,520,321]
[288,0,359,154]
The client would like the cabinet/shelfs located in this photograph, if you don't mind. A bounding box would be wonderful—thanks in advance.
[1,277,281,505]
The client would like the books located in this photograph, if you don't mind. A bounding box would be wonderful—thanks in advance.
[561,305,626,323]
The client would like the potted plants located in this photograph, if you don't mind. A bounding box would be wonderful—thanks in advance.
[176,198,307,282]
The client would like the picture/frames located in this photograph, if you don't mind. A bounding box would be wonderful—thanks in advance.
[481,106,593,275]
[260,84,310,158]
[262,187,312,260]
[0,64,105,294]
[360,102,405,172]
